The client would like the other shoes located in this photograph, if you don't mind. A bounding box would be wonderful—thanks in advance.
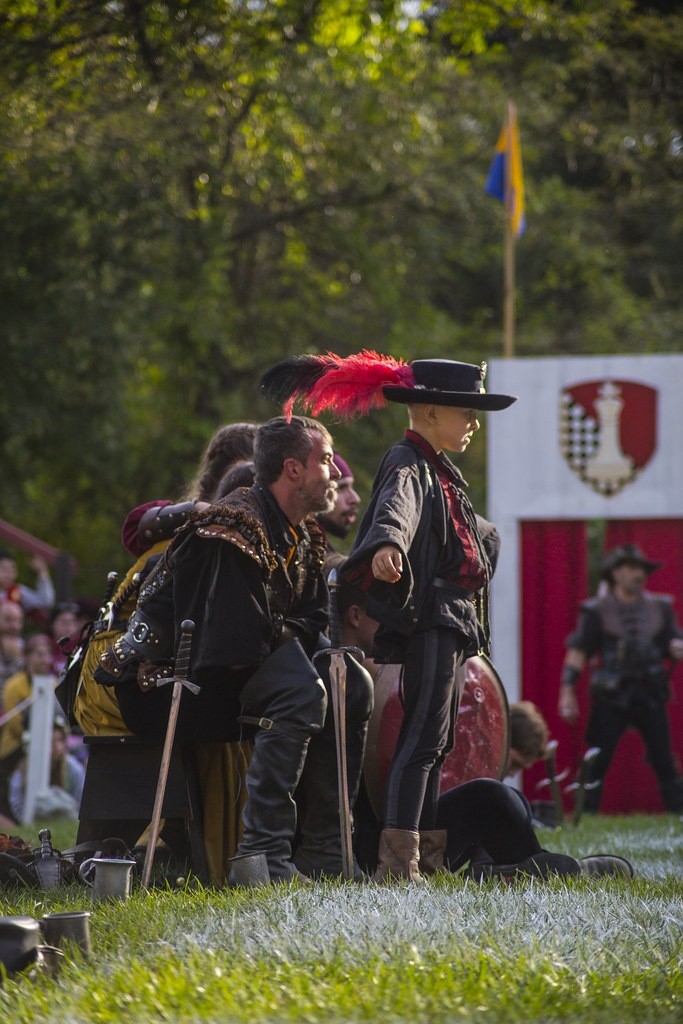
[466,852,585,891]
[570,855,637,884]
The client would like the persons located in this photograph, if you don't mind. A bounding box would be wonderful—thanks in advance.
[511,701,545,783]
[330,570,635,883]
[0,539,96,823]
[343,357,503,886]
[557,544,683,820]
[76,462,260,886]
[311,455,361,579]
[122,422,264,559]
[109,415,378,888]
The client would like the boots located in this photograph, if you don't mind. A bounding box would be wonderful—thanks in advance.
[583,758,613,819]
[644,750,683,818]
[233,638,324,895]
[304,645,377,895]
[377,828,433,896]
[420,826,461,893]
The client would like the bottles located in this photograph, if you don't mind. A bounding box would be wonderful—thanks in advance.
[25,828,74,894]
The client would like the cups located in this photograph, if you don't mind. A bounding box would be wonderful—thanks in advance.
[227,847,269,891]
[79,856,138,907]
[36,911,93,968]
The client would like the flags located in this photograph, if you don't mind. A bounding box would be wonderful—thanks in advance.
[487,107,530,237]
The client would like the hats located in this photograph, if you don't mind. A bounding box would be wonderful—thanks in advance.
[380,355,518,413]
[601,542,667,580]
